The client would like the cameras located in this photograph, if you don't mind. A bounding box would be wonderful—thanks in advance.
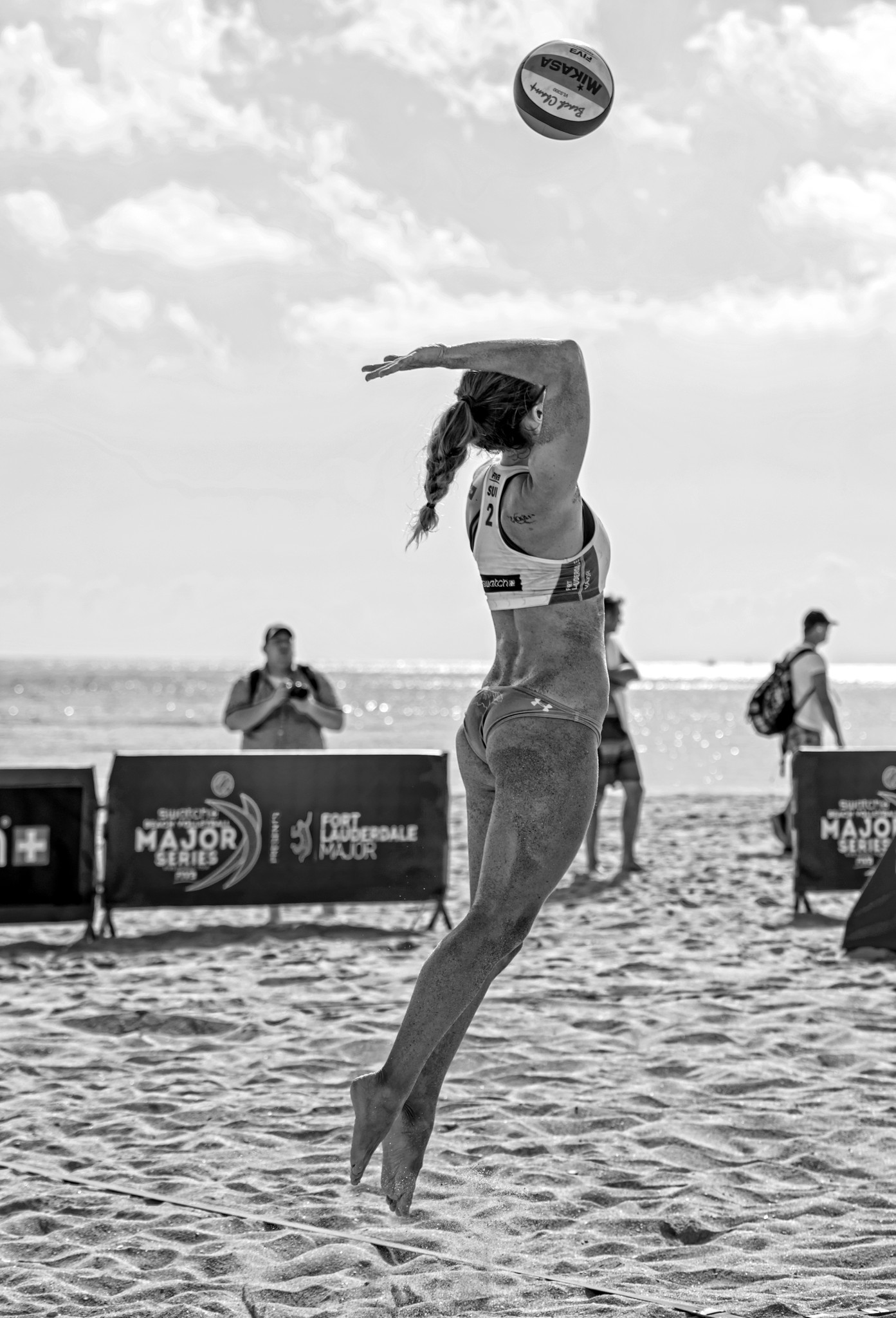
[286,682,308,701]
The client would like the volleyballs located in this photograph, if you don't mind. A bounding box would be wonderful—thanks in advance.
[513,40,615,142]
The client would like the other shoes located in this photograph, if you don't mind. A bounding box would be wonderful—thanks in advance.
[772,812,793,848]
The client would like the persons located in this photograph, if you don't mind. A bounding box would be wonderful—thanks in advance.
[223,624,343,750]
[350,339,610,1216]
[587,598,644,871]
[771,610,843,859]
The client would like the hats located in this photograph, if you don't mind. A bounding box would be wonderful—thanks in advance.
[805,610,838,628]
[604,596,620,611]
[267,626,292,640]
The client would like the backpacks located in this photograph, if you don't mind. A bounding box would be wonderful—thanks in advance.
[748,649,816,735]
